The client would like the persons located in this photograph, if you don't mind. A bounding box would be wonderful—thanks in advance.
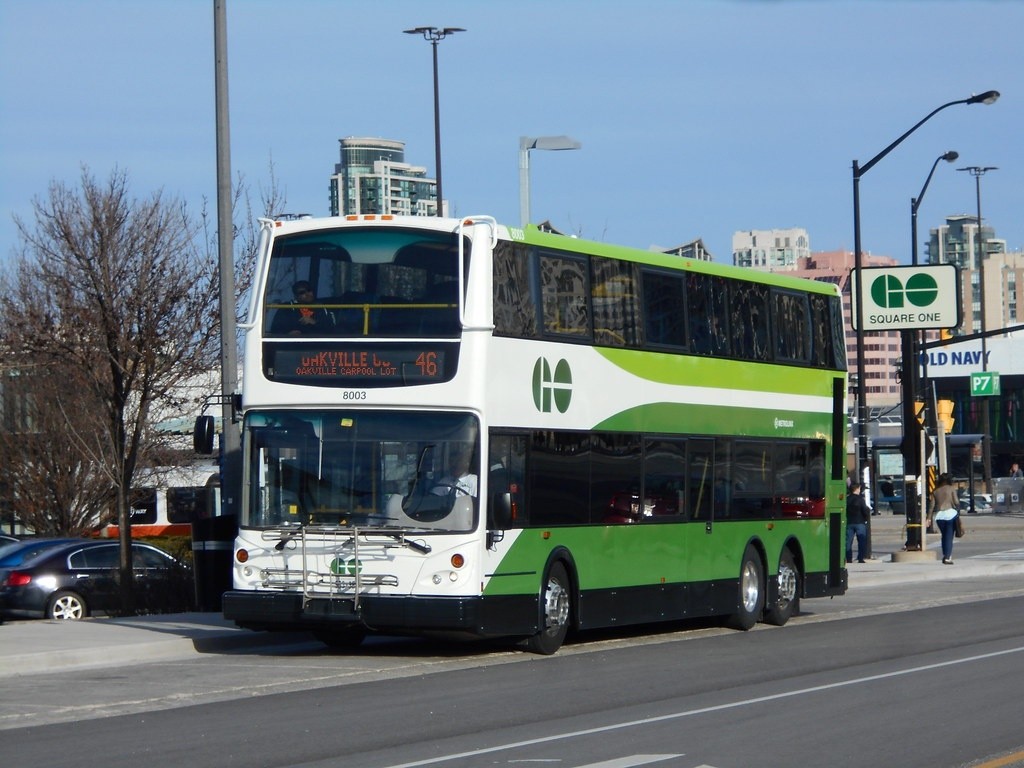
[432,449,477,499]
[1009,464,1023,477]
[925,472,961,565]
[846,482,870,563]
[880,475,896,512]
[271,280,339,338]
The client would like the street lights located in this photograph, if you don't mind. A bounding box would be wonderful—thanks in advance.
[911,150,958,264]
[404,26,469,217]
[852,88,1002,563]
[518,135,582,230]
[955,165,999,333]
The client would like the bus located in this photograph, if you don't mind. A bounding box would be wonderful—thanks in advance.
[0,464,223,613]
[192,214,851,657]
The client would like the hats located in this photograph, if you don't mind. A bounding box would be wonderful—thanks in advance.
[449,444,475,457]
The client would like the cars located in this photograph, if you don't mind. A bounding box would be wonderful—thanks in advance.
[0,537,193,621]
[1,535,21,551]
[960,493,994,516]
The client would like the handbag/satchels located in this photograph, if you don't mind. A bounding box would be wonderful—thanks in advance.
[955,509,964,537]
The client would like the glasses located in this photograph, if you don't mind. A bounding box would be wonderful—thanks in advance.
[296,287,313,295]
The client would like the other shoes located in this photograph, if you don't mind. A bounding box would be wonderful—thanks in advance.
[847,560,852,563]
[942,555,954,564]
[859,560,865,563]
